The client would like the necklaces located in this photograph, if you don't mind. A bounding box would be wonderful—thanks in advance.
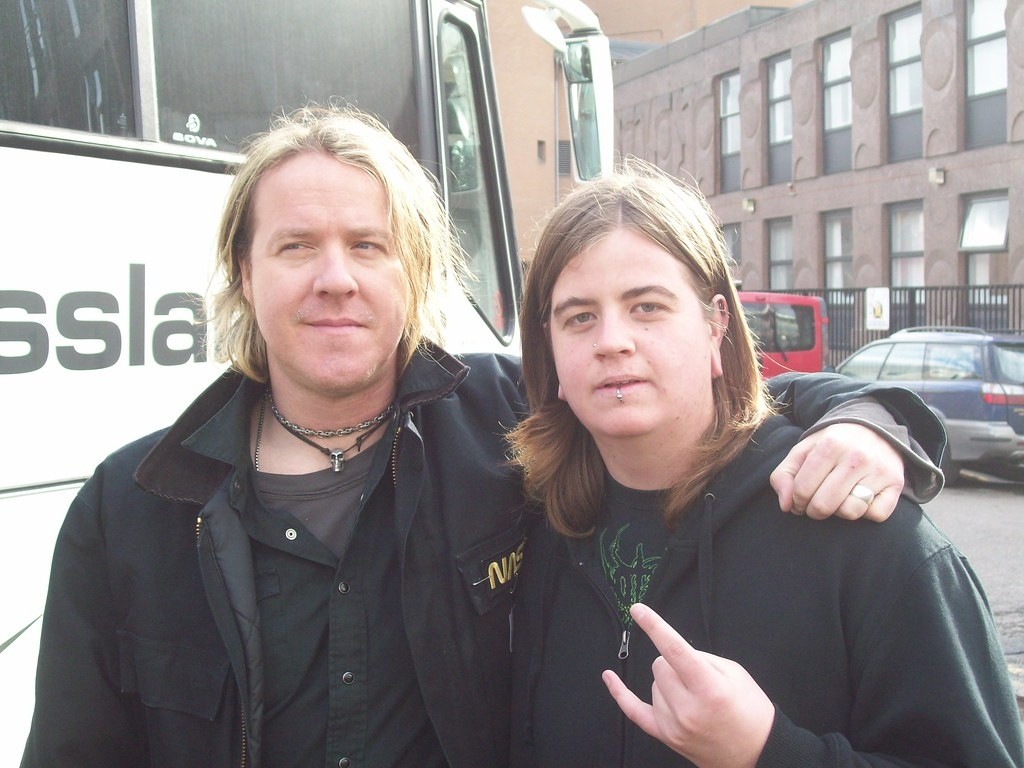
[265,384,397,436]
[254,390,269,473]
[268,404,397,473]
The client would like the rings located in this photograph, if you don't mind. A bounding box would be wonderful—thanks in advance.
[850,485,876,506]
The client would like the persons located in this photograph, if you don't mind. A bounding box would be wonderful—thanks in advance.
[503,168,1024,768]
[20,109,948,767]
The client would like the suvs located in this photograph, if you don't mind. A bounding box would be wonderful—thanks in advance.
[838,323,1024,489]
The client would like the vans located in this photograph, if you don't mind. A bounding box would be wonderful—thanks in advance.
[735,291,834,382]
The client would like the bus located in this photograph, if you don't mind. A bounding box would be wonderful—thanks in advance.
[2,0,618,767]
